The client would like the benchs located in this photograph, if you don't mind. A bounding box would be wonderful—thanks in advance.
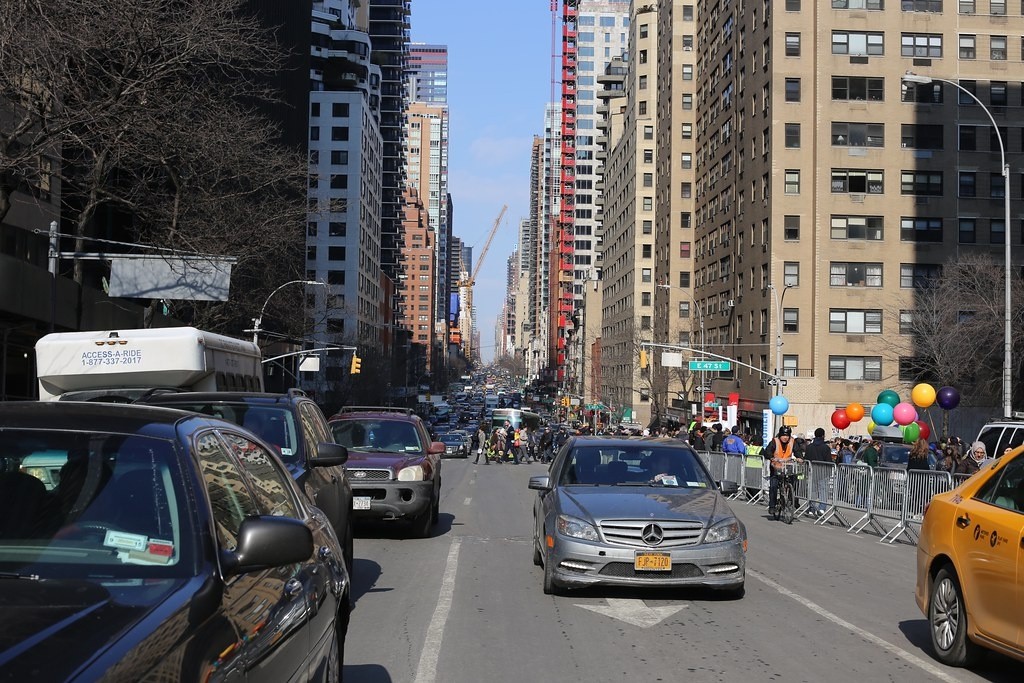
[593,461,646,481]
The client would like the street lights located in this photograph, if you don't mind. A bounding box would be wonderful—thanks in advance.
[902,72,1014,422]
[253,279,323,344]
[657,282,706,417]
[767,282,799,437]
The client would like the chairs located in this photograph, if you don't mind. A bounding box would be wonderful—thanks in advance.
[0,469,52,540]
[573,448,604,484]
[637,455,654,481]
[102,467,191,544]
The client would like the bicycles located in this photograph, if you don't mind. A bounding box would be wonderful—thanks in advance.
[770,457,801,524]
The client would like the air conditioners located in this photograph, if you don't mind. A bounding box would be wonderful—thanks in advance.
[728,300,735,306]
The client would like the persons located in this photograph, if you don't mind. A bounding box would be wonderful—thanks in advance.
[871,403,894,426]
[472,426,489,465]
[795,437,931,512]
[1001,443,1013,456]
[639,452,687,485]
[954,441,987,478]
[805,427,832,515]
[689,423,765,502]
[928,436,965,494]
[490,428,502,463]
[539,426,557,464]
[512,425,532,464]
[554,427,570,452]
[528,427,539,461]
[496,419,519,464]
[764,425,804,514]
[575,426,688,441]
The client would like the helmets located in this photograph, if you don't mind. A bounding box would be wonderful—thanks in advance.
[779,425,792,436]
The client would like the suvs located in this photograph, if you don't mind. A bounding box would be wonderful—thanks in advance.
[329,403,447,539]
[970,411,1024,468]
[854,442,914,510]
[131,386,355,586]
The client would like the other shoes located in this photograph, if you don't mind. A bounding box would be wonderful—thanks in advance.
[513,462,519,465]
[809,512,818,515]
[528,461,532,464]
[496,460,503,463]
[535,459,539,462]
[821,510,824,515]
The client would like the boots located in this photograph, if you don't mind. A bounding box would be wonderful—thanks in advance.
[484,453,489,465]
[472,453,480,464]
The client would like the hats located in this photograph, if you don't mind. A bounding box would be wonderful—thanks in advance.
[1002,443,1014,453]
[751,435,762,444]
[545,427,550,430]
[560,426,566,430]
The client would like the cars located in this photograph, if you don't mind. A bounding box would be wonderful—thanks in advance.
[426,364,583,459]
[0,399,354,683]
[914,443,1024,672]
[527,436,750,602]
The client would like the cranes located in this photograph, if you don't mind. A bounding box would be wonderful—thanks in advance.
[455,205,509,358]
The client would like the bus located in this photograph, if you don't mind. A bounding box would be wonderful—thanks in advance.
[35,325,263,404]
[492,407,542,435]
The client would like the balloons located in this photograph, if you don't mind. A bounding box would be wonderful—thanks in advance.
[893,403,917,425]
[894,384,960,443]
[867,421,875,436]
[831,410,850,430]
[846,402,864,422]
[769,396,789,415]
[877,390,900,407]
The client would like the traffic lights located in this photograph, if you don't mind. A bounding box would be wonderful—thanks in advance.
[561,397,565,406]
[566,397,570,406]
[351,355,361,374]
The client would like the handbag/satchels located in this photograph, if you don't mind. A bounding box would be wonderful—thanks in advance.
[528,441,535,448]
